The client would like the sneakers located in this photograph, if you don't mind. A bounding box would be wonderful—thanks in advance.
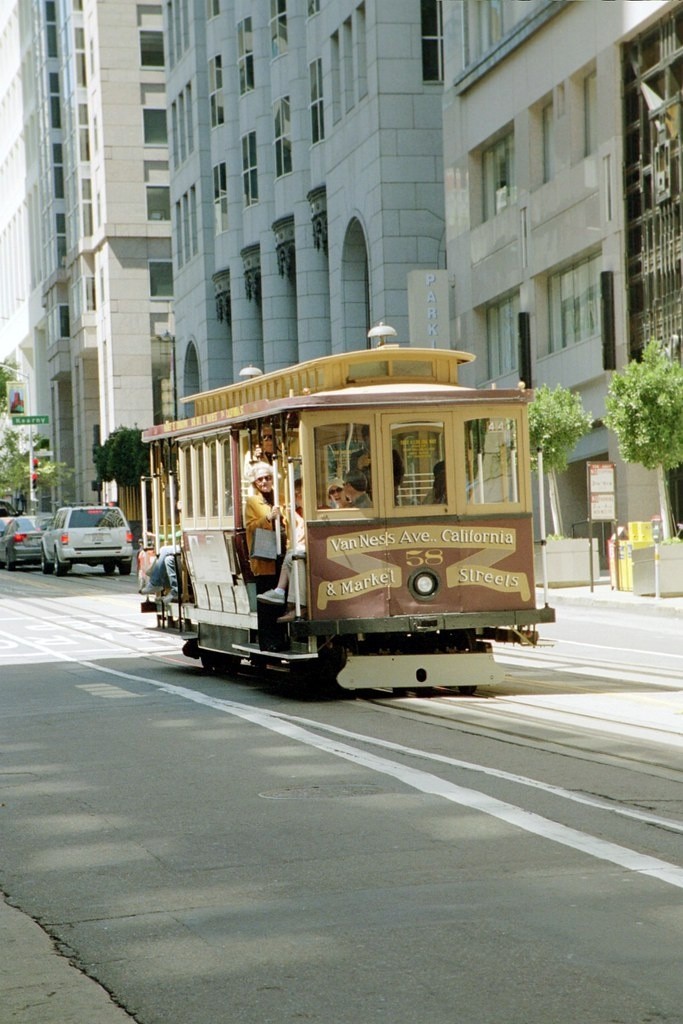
[256,589,285,605]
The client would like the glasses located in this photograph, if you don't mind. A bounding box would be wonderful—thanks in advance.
[256,476,272,482]
[330,487,342,495]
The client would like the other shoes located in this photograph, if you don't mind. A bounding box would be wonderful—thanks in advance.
[139,583,164,594]
[156,593,179,603]
[277,608,307,623]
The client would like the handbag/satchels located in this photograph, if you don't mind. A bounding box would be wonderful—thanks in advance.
[249,528,279,561]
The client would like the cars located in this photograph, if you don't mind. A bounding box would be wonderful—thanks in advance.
[0,499,53,571]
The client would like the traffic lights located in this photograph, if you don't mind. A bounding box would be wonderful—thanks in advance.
[33,458,38,469]
[32,473,37,489]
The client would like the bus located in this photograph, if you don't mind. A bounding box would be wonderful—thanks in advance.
[136,345,557,703]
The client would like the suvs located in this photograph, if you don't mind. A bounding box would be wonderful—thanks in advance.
[40,501,134,576]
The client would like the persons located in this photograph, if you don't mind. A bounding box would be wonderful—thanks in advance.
[350,425,405,506]
[243,427,308,622]
[139,546,183,605]
[423,461,447,504]
[328,470,372,508]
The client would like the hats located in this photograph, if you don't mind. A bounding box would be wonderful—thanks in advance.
[249,462,272,482]
[327,480,344,493]
